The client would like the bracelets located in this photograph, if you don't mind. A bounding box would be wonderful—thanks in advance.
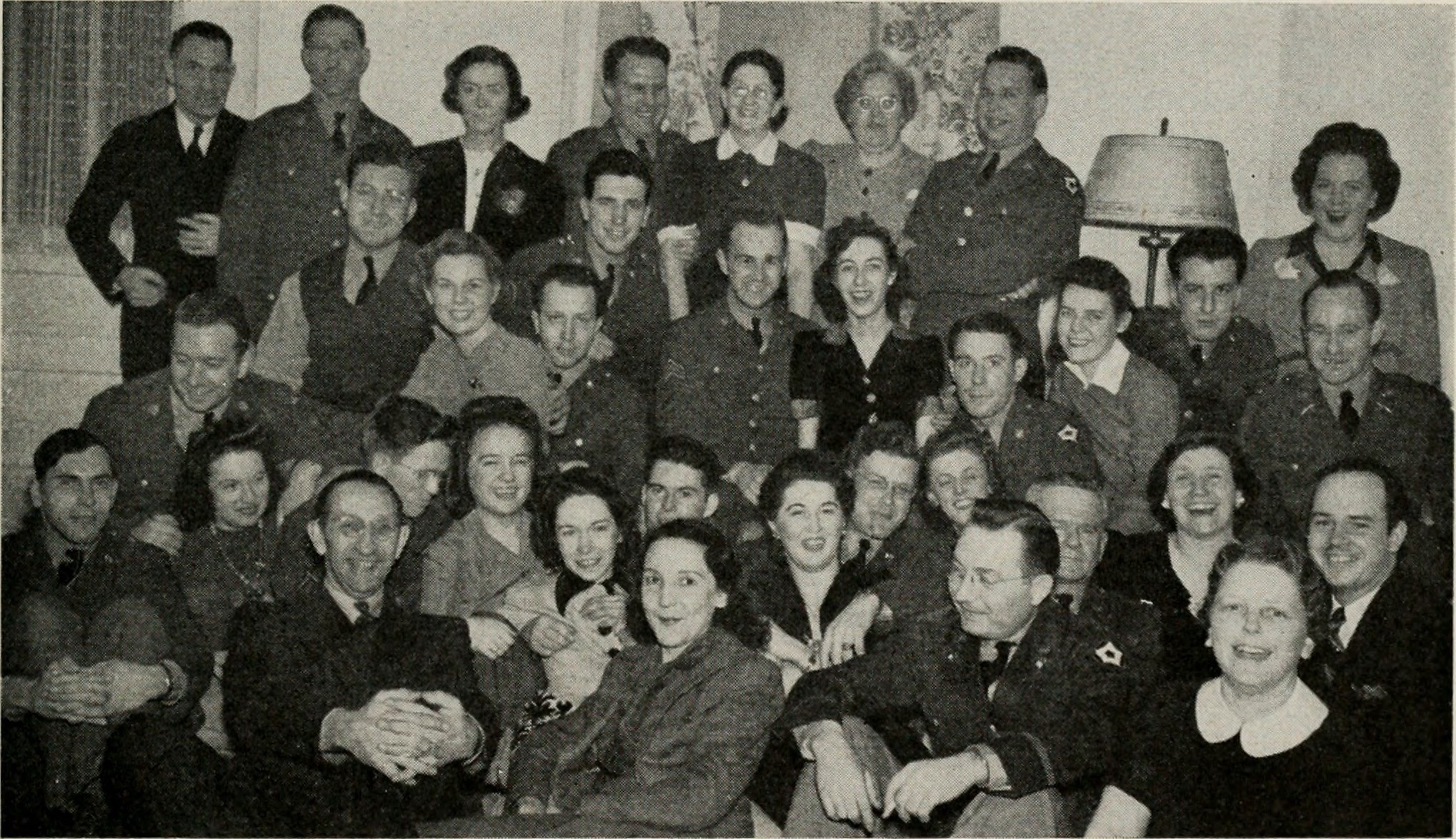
[801,650,817,672]
[153,661,174,702]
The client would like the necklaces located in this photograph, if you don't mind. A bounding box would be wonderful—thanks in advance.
[208,516,264,591]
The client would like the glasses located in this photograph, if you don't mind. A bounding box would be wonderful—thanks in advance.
[944,559,1039,592]
[729,82,775,101]
[393,459,448,484]
[856,96,904,113]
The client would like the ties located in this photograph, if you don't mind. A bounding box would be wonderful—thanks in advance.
[636,136,656,175]
[56,549,85,587]
[981,640,1017,703]
[850,538,871,573]
[599,261,616,305]
[750,315,763,351]
[355,252,380,307]
[351,601,380,657]
[982,150,1002,181]
[1337,389,1362,443]
[330,111,348,160]
[184,125,205,167]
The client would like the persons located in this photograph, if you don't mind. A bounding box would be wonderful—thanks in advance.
[0,6,1453,838]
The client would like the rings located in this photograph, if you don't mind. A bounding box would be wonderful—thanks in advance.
[490,646,497,651]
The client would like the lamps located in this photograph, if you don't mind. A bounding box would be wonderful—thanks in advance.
[1083,118,1241,307]
